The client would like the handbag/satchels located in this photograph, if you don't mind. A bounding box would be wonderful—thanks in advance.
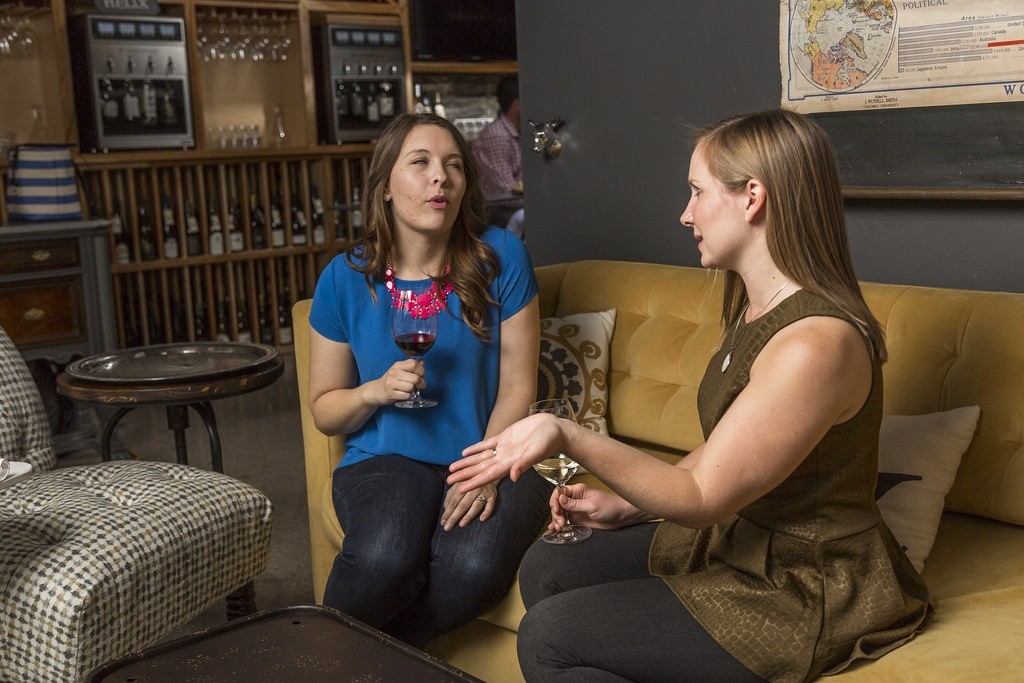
[7,144,91,224]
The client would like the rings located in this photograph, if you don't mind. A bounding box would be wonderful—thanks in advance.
[494,448,497,455]
[478,495,487,502]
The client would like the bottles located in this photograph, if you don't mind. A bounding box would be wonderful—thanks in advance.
[108,182,369,349]
[413,82,448,120]
[334,79,397,130]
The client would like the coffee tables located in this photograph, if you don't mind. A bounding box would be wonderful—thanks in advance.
[86,603,487,683]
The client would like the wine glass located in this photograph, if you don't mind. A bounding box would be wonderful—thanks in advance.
[386,287,439,408]
[196,7,294,62]
[528,399,593,546]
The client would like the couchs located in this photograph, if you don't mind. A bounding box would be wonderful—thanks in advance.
[292,261,1023,683]
[0,329,274,683]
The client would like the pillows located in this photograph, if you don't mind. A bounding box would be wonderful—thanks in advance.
[525,309,614,471]
[877,405,982,573]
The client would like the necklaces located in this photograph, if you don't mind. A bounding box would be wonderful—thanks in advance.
[384,248,453,319]
[722,280,790,373]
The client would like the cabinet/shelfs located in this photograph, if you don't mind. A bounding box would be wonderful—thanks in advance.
[79,141,382,341]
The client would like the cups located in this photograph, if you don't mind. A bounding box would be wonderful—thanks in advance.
[203,110,288,150]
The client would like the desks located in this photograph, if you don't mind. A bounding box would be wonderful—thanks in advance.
[1,214,137,462]
[54,341,284,474]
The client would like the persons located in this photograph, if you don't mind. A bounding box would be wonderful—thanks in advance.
[470,72,524,200]
[447,110,937,683]
[308,113,557,650]
